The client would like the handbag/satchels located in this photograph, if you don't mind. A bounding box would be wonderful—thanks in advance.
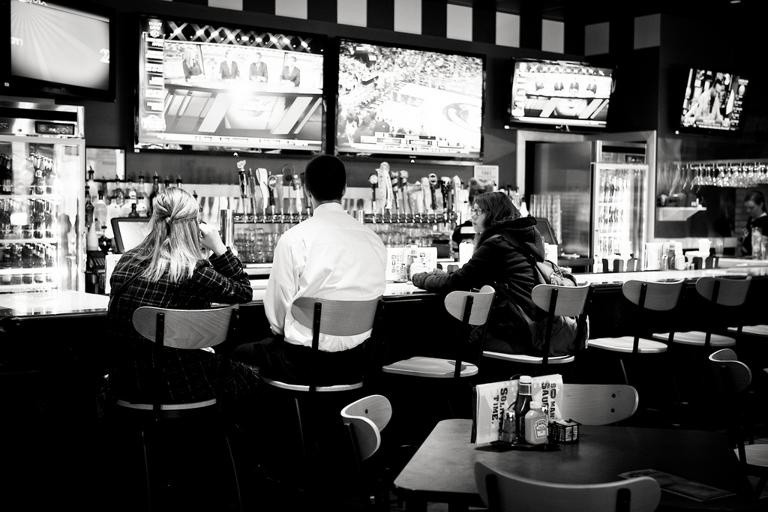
[529,258,589,357]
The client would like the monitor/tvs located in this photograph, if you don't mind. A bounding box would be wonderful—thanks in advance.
[325,32,488,164]
[677,65,752,136]
[1,1,117,102]
[508,55,619,132]
[129,21,332,157]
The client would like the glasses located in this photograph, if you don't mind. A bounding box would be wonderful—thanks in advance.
[470,209,487,215]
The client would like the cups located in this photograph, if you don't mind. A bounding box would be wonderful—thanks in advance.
[760,236,768,260]
[236,226,276,265]
[597,172,628,256]
[751,227,762,260]
[529,193,564,245]
[375,224,441,247]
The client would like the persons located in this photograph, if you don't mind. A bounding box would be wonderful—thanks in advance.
[451,175,498,262]
[181,45,202,80]
[534,79,598,93]
[682,79,736,129]
[338,43,484,146]
[409,191,590,358]
[279,55,300,86]
[102,187,254,512]
[235,153,388,388]
[247,51,268,81]
[736,191,768,258]
[219,49,240,80]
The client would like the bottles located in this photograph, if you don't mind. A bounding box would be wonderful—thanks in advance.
[501,410,516,443]
[515,374,533,443]
[706,248,718,269]
[0,155,60,294]
[84,173,184,253]
[524,402,550,444]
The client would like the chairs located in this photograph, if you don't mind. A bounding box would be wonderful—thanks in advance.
[483,283,593,380]
[339,394,393,512]
[652,276,752,379]
[114,305,242,512]
[250,296,382,475]
[706,349,768,512]
[473,461,661,512]
[727,325,768,375]
[382,285,495,468]
[587,279,685,388]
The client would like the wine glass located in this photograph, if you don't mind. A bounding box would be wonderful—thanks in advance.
[682,163,768,189]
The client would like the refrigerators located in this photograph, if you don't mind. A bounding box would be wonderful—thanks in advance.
[535,141,647,274]
[0,99,88,298]
